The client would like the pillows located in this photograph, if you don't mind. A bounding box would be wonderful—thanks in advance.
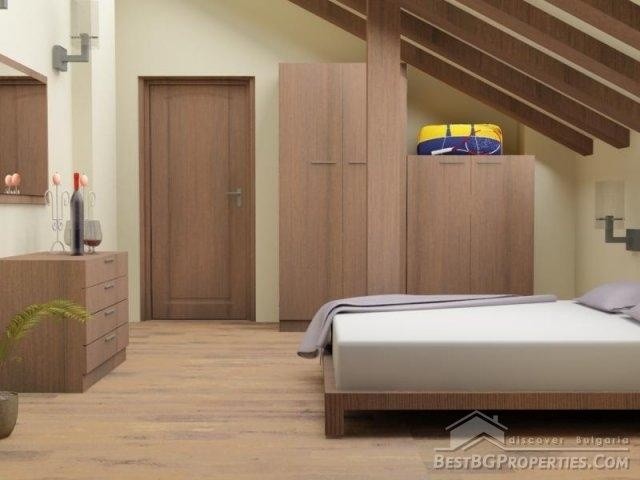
[621,304,639,322]
[572,281,639,313]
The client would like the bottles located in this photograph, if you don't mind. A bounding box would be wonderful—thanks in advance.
[69,173,85,257]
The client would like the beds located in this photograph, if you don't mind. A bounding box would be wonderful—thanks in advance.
[297,291,639,438]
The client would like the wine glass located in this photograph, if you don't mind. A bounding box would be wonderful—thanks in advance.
[85,219,102,255]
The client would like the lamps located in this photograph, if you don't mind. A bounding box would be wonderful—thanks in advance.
[51,0,101,72]
[594,180,639,253]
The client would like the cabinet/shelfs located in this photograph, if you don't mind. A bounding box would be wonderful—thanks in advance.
[0,251,129,392]
[407,154,536,294]
[277,61,408,332]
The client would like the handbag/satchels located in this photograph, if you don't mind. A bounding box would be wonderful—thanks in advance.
[417,121,506,158]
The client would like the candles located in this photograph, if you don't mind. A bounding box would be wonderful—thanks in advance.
[4,175,13,187]
[53,173,60,185]
[81,175,88,185]
[12,173,21,185]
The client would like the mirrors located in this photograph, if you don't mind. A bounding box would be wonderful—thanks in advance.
[0,51,50,205]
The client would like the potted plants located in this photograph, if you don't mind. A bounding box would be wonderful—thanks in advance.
[0,298,95,439]
